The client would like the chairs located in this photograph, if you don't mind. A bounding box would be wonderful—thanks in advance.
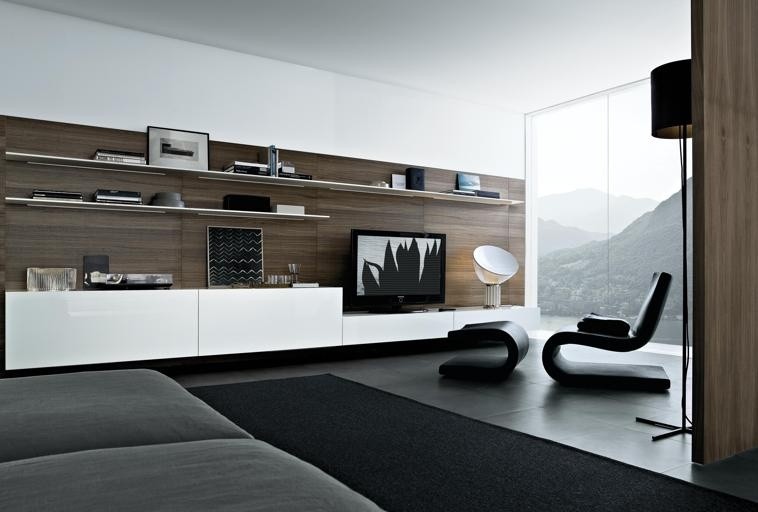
[543,272,671,393]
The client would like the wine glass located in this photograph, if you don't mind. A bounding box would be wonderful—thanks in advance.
[288,263,301,284]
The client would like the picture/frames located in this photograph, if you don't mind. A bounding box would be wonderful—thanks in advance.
[147,125,209,171]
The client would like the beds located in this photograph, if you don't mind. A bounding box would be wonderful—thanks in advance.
[1,369,254,462]
[1,439,383,511]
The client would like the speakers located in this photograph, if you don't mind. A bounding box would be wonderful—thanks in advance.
[406,168,424,190]
[83,254,110,290]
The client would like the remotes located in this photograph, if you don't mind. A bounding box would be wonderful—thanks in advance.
[438,308,456,312]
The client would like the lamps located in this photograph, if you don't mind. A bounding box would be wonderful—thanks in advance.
[634,59,694,443]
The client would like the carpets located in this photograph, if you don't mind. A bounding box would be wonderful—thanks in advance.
[186,372,758,512]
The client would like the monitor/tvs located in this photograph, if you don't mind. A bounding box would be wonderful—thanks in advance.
[350,229,446,313]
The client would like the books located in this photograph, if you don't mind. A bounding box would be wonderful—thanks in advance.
[92,155,147,166]
[278,172,313,180]
[93,188,141,198]
[94,195,140,202]
[95,149,145,158]
[229,169,269,176]
[95,153,145,161]
[92,198,144,206]
[473,190,501,198]
[442,189,476,196]
[222,160,268,168]
[222,165,270,173]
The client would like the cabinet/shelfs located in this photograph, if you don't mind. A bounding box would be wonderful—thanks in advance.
[3,149,524,218]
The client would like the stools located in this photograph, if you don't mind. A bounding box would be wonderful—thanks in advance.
[439,321,530,383]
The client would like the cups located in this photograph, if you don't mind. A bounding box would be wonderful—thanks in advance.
[268,274,291,284]
